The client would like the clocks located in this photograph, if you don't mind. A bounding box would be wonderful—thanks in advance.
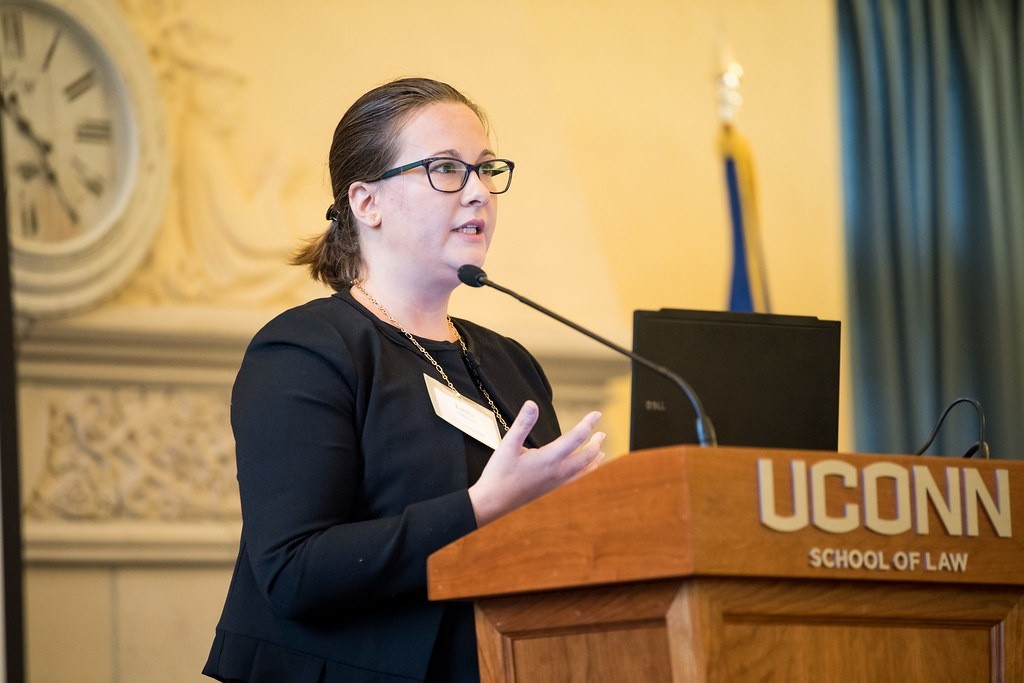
[0,0,169,320]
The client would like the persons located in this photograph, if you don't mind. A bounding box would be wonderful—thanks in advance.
[201,77,607,683]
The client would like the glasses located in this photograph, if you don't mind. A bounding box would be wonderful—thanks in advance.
[365,157,514,194]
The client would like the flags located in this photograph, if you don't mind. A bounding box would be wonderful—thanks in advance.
[717,125,769,312]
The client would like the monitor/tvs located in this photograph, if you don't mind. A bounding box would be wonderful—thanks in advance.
[629,307,843,453]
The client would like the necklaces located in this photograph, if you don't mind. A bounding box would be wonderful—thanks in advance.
[351,278,509,432]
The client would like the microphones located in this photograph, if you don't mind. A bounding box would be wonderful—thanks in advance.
[458,265,717,448]
